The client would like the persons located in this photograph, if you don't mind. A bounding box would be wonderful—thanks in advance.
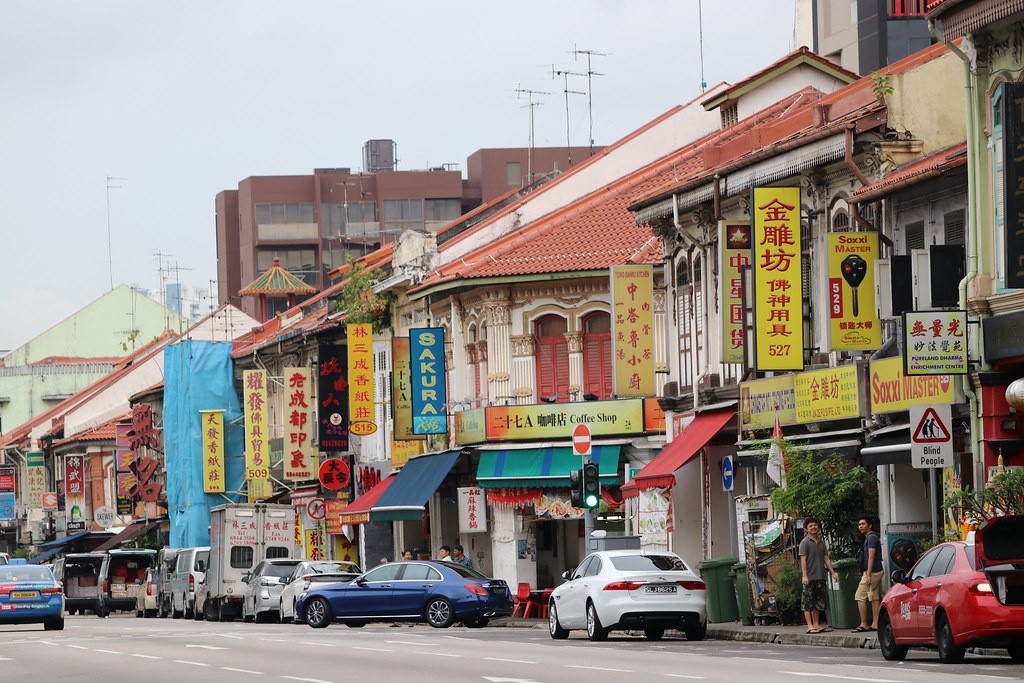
[799,518,839,634]
[438,543,472,627]
[389,550,416,627]
[851,518,885,633]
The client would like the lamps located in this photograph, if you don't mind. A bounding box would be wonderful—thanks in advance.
[583,392,598,401]
[885,127,898,140]
[540,393,558,403]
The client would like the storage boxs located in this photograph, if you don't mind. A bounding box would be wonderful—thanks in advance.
[67,585,79,597]
[110,583,126,591]
[113,576,125,583]
[111,591,126,599]
[67,576,78,585]
[80,575,96,586]
[79,586,98,596]
[126,584,141,598]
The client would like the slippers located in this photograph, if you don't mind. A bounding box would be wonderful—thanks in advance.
[867,626,877,631]
[851,626,870,633]
[819,627,834,632]
[805,628,822,634]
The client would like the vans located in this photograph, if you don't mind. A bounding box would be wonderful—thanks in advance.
[50,547,182,618]
[166,546,211,620]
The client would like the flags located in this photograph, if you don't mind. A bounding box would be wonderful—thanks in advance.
[766,418,791,491]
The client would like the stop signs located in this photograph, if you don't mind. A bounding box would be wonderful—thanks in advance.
[572,425,592,455]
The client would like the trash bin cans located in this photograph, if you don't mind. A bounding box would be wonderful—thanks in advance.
[732,561,755,625]
[698,556,740,623]
[822,558,862,629]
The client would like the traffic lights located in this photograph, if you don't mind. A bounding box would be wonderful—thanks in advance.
[569,469,585,509]
[584,462,600,509]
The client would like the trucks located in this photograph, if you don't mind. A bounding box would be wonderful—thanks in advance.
[203,502,294,625]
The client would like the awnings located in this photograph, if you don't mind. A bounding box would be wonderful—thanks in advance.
[618,478,639,500]
[26,548,65,564]
[634,407,737,491]
[475,444,629,488]
[92,519,160,552]
[339,449,462,525]
[37,530,90,547]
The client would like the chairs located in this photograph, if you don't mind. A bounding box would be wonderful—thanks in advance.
[542,587,557,619]
[512,582,541,619]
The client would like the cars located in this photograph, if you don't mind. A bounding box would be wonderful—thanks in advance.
[0,558,66,630]
[295,559,515,631]
[547,548,710,642]
[240,558,308,624]
[278,562,363,624]
[876,514,1024,664]
[0,552,12,566]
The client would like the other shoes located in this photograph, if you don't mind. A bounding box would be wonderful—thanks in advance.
[455,622,463,627]
[409,623,414,627]
[389,624,402,627]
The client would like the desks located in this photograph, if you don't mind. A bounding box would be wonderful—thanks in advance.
[530,589,554,617]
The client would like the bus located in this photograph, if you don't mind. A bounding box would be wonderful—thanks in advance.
[191,576,205,620]
